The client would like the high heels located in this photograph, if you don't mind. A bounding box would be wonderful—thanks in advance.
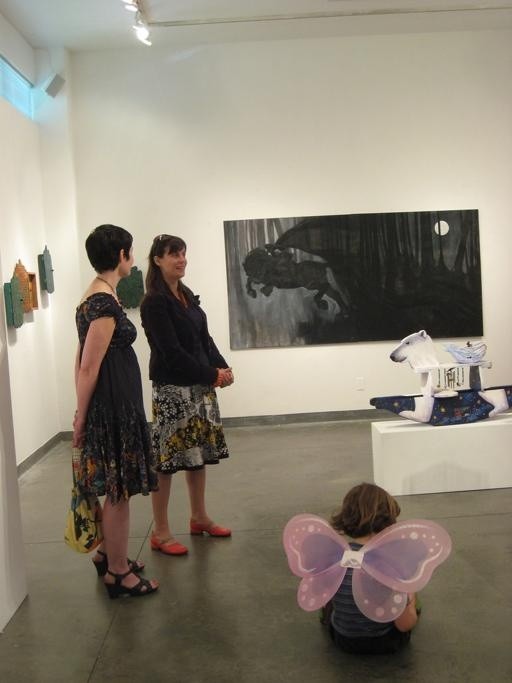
[149,529,188,556]
[91,548,145,575]
[188,516,231,538]
[102,568,158,598]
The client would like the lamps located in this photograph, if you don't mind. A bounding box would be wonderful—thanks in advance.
[122,0,152,48]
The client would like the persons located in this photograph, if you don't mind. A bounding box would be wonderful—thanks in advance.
[67,222,162,601]
[298,483,420,655]
[140,234,233,557]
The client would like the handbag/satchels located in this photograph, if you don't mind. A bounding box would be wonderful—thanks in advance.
[61,475,104,554]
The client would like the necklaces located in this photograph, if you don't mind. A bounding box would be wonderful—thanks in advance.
[95,277,117,297]
